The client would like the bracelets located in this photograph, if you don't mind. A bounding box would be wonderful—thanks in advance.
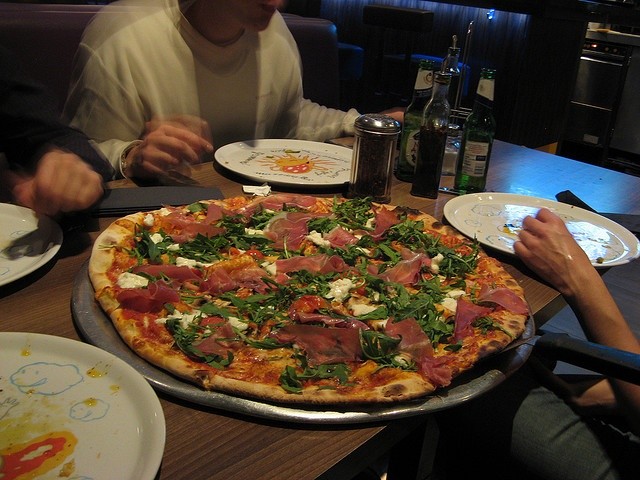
[121,143,138,176]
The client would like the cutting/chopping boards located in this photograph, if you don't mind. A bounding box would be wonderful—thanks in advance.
[71,257,639,425]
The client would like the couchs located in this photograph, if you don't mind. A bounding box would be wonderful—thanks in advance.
[1,4,365,108]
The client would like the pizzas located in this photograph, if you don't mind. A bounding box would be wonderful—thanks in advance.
[89,193,528,404]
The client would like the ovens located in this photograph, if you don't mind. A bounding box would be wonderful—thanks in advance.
[505,0,639,176]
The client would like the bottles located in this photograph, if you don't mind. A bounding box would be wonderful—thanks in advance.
[441,34,463,121]
[344,113,401,204]
[394,59,437,182]
[410,53,454,200]
[452,65,496,195]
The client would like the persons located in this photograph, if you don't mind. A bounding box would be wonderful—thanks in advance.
[1,112,113,216]
[440,206,639,479]
[57,0,404,183]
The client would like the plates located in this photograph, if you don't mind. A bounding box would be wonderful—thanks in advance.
[0,331,165,480]
[443,191,640,268]
[212,138,354,188]
[1,203,65,287]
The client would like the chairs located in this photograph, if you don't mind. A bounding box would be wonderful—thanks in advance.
[525,327,638,479]
